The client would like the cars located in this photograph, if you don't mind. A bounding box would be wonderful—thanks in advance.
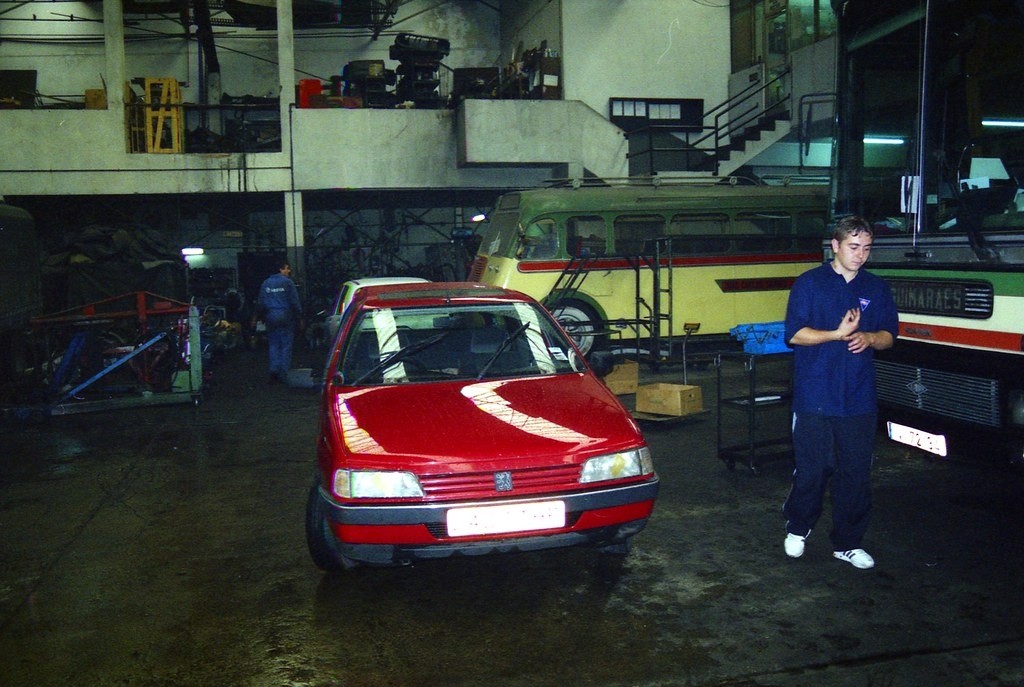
[306,281,659,575]
[316,277,453,355]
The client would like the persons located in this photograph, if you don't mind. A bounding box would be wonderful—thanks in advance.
[257,263,302,381]
[784,216,899,569]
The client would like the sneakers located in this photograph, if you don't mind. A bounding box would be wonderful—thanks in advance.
[834,549,874,568]
[784,533,806,558]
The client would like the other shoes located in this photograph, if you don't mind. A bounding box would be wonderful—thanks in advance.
[279,368,288,378]
[272,365,280,375]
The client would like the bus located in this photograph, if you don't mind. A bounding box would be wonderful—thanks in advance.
[467,180,832,363]
[799,0,1024,474]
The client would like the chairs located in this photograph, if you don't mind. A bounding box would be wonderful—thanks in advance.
[340,328,425,383]
[587,234,601,253]
[451,328,523,371]
[363,325,449,345]
